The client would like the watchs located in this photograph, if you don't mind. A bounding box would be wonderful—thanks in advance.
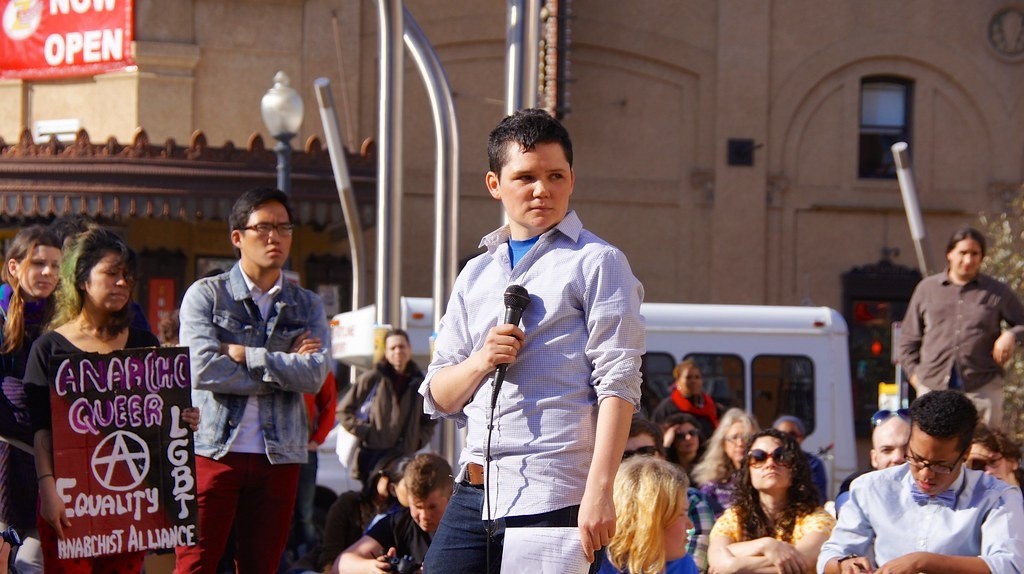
[837,553,858,574]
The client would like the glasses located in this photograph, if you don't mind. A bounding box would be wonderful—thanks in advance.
[241,222,297,236]
[622,445,655,460]
[673,428,701,440]
[964,455,1004,473]
[903,441,970,474]
[787,431,804,439]
[870,408,913,430]
[747,446,795,469]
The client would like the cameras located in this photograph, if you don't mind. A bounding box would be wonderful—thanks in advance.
[386,555,422,574]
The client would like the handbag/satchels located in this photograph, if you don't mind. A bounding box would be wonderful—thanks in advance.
[336,399,371,478]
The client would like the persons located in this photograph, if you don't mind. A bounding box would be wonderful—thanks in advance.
[656,414,705,476]
[0,215,77,574]
[336,328,436,486]
[22,229,200,574]
[418,109,646,574]
[690,410,762,519]
[896,228,1024,432]
[172,188,333,574]
[707,429,836,574]
[651,360,725,439]
[623,418,714,574]
[815,391,1024,574]
[964,422,1024,492]
[835,408,915,520]
[333,453,455,574]
[773,416,829,507]
[593,454,700,574]
[0,536,14,574]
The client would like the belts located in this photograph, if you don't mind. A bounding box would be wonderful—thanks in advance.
[461,462,487,487]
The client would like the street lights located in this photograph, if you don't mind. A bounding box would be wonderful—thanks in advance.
[260,69,304,271]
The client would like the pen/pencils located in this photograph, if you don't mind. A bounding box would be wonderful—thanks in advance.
[853,562,865,570]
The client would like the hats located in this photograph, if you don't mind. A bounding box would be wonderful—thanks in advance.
[772,415,805,437]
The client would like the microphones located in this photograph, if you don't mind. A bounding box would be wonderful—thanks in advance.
[490,285,530,407]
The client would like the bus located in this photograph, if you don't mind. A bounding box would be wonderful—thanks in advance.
[313,294,858,527]
